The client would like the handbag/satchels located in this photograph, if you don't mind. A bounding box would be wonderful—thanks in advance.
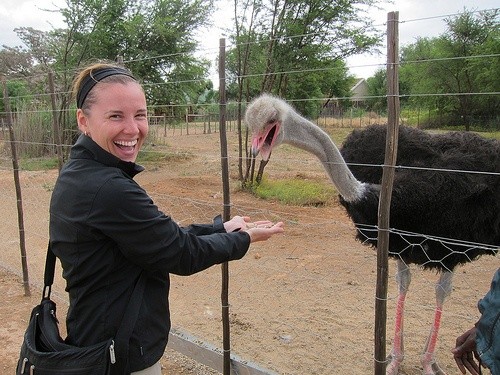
[16,299,125,375]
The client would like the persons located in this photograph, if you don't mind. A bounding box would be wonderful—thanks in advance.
[450,269,500,375]
[48,63,284,375]
